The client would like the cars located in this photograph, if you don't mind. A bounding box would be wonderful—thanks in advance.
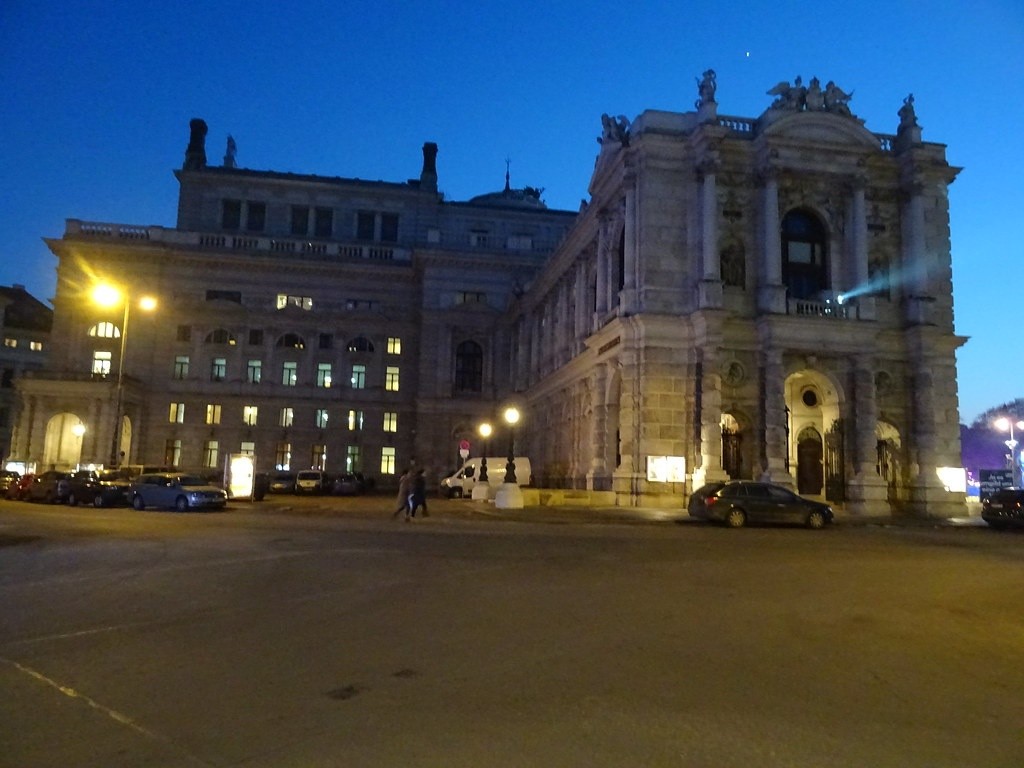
[337,472,377,497]
[687,479,834,529]
[981,486,1024,529]
[268,471,295,494]
[295,470,323,495]
[57,470,132,507]
[4,473,36,501]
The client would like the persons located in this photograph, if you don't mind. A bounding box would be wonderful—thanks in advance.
[391,469,412,522]
[411,470,430,518]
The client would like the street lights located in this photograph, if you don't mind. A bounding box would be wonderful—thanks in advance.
[478,423,493,481]
[994,417,1024,486]
[93,280,158,471]
[504,408,519,483]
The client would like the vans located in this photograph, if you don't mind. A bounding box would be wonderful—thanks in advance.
[440,456,534,499]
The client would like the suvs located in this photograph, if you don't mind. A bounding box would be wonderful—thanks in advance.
[0,470,21,493]
[127,472,228,512]
[30,469,72,504]
